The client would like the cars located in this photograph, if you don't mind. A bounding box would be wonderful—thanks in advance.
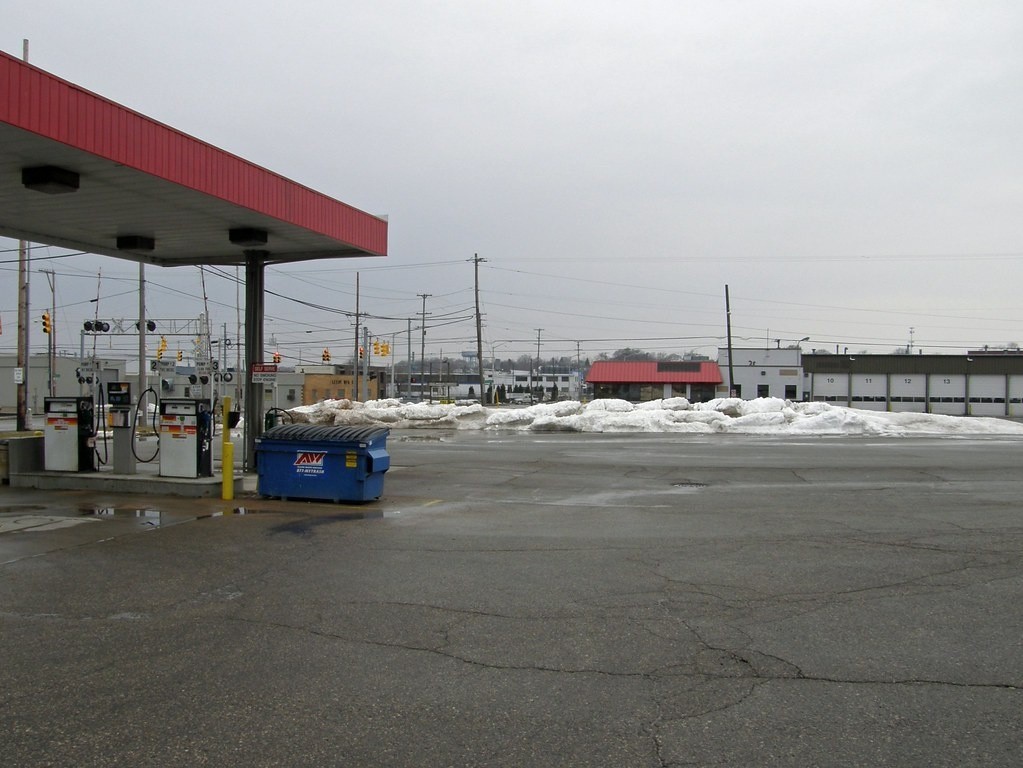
[513,395,539,404]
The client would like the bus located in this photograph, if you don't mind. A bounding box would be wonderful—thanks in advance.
[388,373,487,398]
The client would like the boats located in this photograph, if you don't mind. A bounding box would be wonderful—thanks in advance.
[380,344,391,356]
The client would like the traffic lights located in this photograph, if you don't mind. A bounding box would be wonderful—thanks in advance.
[178,351,183,361]
[86,378,92,383]
[322,349,331,361]
[42,314,50,333]
[157,348,163,359]
[200,376,208,385]
[360,348,364,359]
[161,340,167,351]
[187,375,197,384]
[374,342,380,354]
[273,352,281,363]
